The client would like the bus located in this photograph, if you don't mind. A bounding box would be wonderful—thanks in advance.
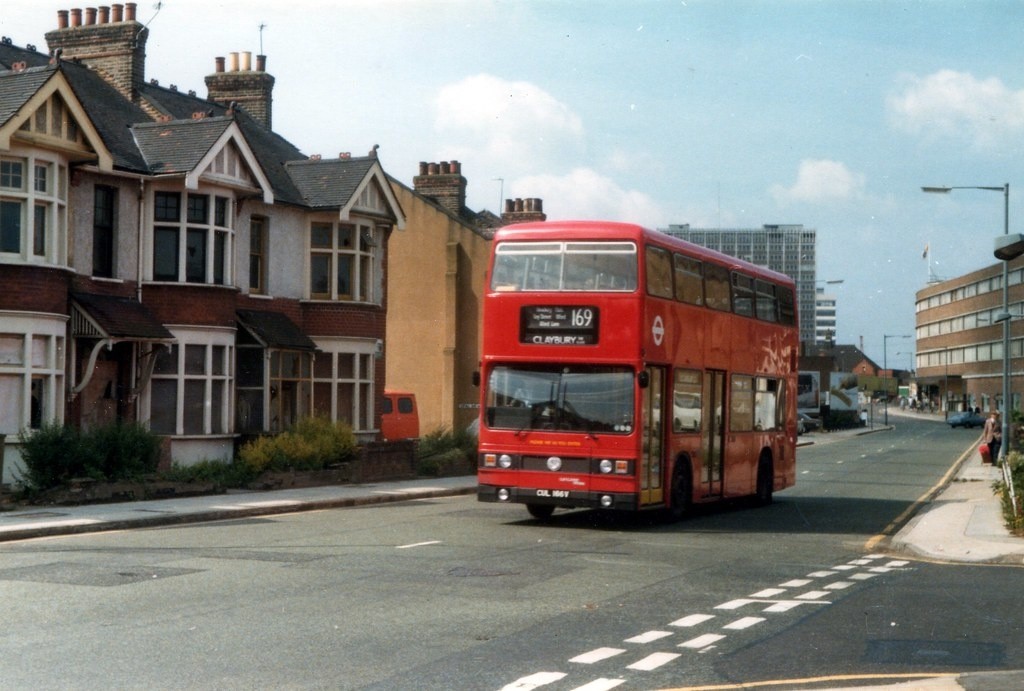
[474,218,800,526]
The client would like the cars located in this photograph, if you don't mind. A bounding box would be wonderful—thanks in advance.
[651,389,710,434]
[946,410,988,429]
[796,412,822,436]
[715,391,776,433]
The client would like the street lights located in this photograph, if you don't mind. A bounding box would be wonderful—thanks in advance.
[896,351,914,399]
[921,345,949,419]
[883,335,914,426]
[918,181,1013,463]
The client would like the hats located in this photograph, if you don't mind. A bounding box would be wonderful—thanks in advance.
[990,411,997,414]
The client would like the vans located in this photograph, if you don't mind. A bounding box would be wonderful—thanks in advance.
[381,388,421,442]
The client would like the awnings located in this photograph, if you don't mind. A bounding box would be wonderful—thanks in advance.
[69,292,179,350]
[236,310,319,350]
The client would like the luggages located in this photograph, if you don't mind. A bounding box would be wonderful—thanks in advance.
[979,442,993,466]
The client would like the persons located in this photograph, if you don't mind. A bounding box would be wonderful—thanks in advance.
[911,398,934,413]
[983,411,1002,466]
[508,380,533,409]
